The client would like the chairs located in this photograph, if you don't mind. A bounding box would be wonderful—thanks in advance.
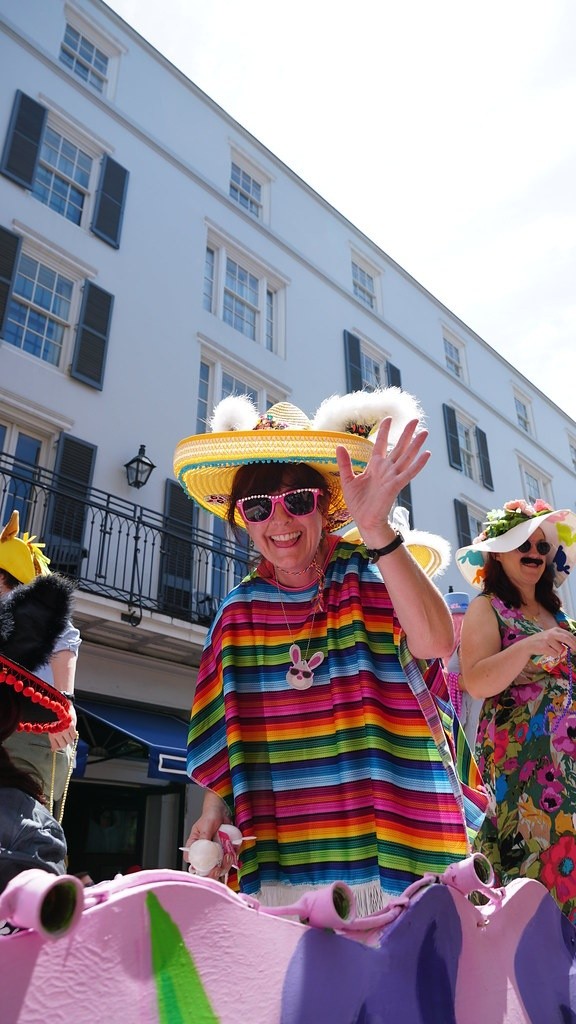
[44,543,88,579]
[193,592,223,627]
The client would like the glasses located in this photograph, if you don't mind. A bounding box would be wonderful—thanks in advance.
[516,540,551,555]
[235,488,325,524]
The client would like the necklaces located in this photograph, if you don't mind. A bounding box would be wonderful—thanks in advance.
[447,669,461,716]
[273,568,325,693]
[553,645,573,729]
[524,601,540,621]
[49,730,79,825]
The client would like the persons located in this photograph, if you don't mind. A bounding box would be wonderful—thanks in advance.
[0,575,72,892]
[439,592,486,755]
[341,506,449,584]
[0,509,84,816]
[455,498,576,932]
[171,385,490,934]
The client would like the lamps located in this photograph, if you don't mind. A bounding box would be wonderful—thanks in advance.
[125,444,157,489]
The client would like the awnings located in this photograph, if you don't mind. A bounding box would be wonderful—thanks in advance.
[71,695,195,782]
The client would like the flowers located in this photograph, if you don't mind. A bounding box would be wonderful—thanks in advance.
[472,498,556,543]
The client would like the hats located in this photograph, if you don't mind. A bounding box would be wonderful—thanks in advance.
[455,500,576,592]
[0,787,67,876]
[0,510,53,584]
[0,573,76,734]
[444,592,470,614]
[172,383,427,531]
[342,506,452,580]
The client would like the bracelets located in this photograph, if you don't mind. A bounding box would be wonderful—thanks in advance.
[58,690,75,699]
[365,530,405,564]
